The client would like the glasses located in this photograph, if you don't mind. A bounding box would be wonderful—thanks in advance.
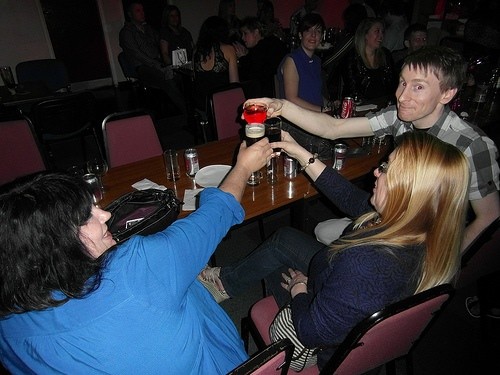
[379,162,388,173]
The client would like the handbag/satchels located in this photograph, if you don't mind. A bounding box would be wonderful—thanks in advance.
[268,304,319,372]
[102,185,185,244]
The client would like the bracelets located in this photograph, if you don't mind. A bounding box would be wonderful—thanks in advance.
[301,153,319,171]
[321,105,323,112]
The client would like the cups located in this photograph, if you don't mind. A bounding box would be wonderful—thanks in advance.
[164,149,181,181]
[264,117,281,152]
[243,102,268,124]
[267,156,281,186]
[248,170,261,186]
[361,136,376,151]
[66,160,85,180]
[321,95,332,116]
[244,122,265,147]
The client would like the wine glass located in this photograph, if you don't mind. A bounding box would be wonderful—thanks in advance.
[86,158,110,194]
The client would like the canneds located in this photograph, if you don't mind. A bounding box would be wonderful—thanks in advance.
[285,179,297,199]
[340,97,353,119]
[284,155,297,178]
[82,173,102,205]
[333,144,346,170]
[183,149,199,175]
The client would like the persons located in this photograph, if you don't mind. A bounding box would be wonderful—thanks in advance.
[0,137,276,375]
[241,44,500,251]
[120,0,500,126]
[197,130,472,371]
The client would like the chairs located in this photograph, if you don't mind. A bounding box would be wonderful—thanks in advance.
[0,51,500,375]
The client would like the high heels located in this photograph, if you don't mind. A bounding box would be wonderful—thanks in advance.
[197,265,230,303]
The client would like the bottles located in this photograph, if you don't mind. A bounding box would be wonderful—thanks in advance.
[463,65,500,126]
[0,65,16,96]
[285,17,343,53]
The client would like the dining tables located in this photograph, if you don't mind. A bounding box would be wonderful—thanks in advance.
[97,110,392,220]
[0,81,61,108]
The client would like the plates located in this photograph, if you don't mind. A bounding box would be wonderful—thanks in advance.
[194,164,232,188]
